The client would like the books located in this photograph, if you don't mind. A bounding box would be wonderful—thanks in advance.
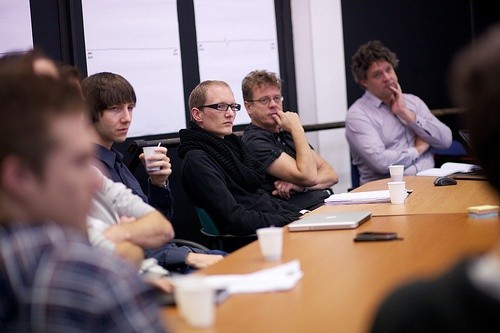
[416,162,481,180]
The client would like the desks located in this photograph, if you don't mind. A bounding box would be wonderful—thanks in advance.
[159,213,500,333]
[299,169,500,219]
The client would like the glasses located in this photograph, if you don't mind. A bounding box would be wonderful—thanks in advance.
[198,103,241,111]
[247,96,283,104]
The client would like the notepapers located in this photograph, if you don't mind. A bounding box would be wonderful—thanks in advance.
[467,204,499,219]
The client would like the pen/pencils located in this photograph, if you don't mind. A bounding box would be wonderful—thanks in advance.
[454,177,487,181]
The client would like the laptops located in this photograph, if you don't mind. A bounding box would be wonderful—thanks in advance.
[287,211,372,228]
[445,130,487,180]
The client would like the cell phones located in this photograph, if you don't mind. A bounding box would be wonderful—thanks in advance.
[354,232,396,241]
[161,289,226,307]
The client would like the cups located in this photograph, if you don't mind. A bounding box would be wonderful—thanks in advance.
[256,227,283,260]
[389,165,405,181]
[389,182,404,205]
[173,277,217,326]
[143,147,162,171]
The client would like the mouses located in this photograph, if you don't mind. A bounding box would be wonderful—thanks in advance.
[434,177,457,186]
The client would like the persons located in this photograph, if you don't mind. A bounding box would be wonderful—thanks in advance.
[1,50,174,278]
[0,71,164,333]
[346,40,452,188]
[170,80,300,254]
[82,72,226,273]
[371,240,500,333]
[240,70,339,211]
